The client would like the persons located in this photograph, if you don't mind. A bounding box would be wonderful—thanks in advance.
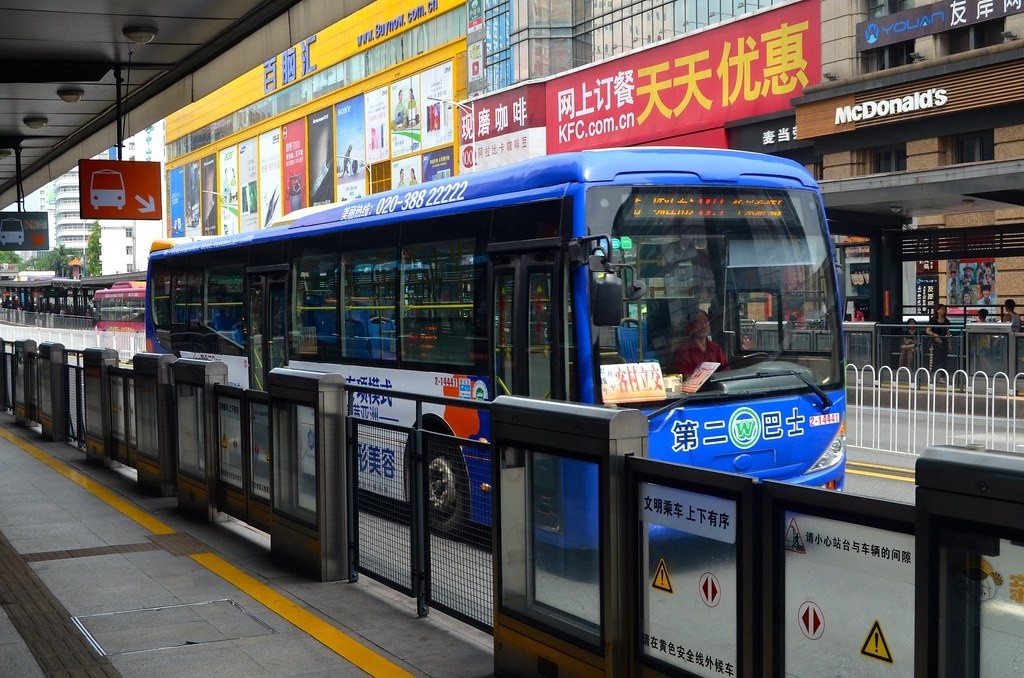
[787,299,1020,371]
[948,261,993,307]
[670,304,731,380]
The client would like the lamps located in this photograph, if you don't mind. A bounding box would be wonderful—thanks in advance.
[123,26,160,46]
[1000,29,1018,42]
[24,116,48,131]
[822,72,837,81]
[56,90,85,104]
[890,206,903,213]
[0,150,11,160]
[962,199,975,207]
[910,52,926,63]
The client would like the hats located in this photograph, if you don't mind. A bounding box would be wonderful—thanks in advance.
[985,263,992,268]
[963,266,974,272]
[981,285,991,291]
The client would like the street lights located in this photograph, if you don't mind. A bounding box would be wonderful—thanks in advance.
[335,154,373,196]
[427,96,476,172]
[202,188,231,236]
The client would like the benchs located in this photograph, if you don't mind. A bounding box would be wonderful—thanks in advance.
[892,335,966,374]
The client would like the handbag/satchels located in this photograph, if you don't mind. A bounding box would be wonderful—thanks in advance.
[901,343,914,350]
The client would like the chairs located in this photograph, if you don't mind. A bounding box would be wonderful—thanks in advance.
[173,282,397,361]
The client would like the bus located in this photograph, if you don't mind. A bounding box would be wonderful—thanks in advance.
[91,280,194,340]
[144,145,848,553]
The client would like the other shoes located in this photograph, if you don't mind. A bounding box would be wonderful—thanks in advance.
[909,367,913,372]
[939,380,947,384]
